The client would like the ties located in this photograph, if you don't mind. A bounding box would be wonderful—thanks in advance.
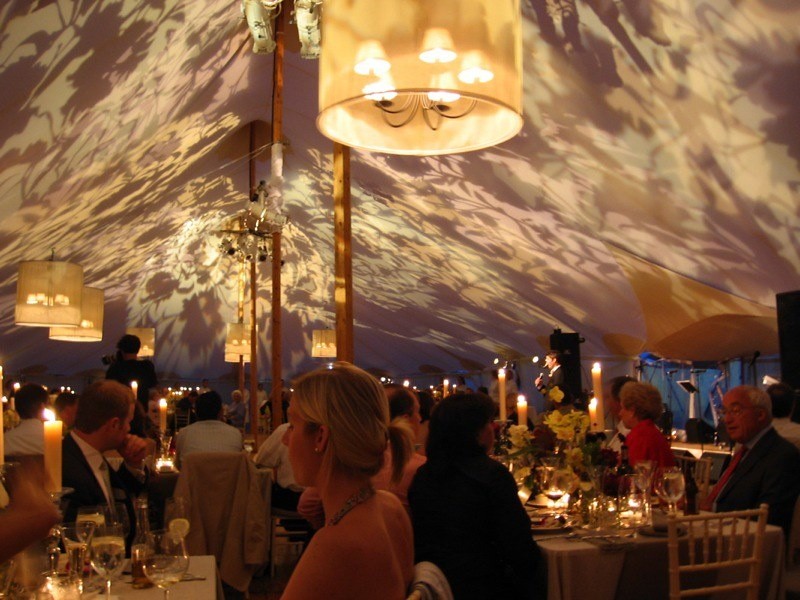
[704,447,746,512]
[99,462,117,519]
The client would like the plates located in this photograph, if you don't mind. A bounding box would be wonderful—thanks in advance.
[527,500,547,507]
[530,516,571,532]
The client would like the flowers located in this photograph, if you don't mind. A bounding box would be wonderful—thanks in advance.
[504,411,616,493]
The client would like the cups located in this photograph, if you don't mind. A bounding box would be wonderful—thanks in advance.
[501,461,514,477]
[618,474,652,528]
[37,570,83,600]
[98,503,131,538]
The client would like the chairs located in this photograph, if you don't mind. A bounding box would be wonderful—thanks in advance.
[675,453,715,510]
[269,506,307,578]
[668,502,771,600]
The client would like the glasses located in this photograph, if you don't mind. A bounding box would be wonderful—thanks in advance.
[722,403,758,416]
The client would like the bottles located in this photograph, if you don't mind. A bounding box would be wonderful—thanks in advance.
[617,445,635,496]
[131,497,156,589]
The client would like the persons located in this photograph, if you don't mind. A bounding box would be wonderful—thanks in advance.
[4,383,48,455]
[298,384,426,531]
[54,392,78,436]
[279,361,415,600]
[226,379,289,427]
[174,391,242,470]
[407,392,545,600]
[535,350,566,412]
[606,376,637,453]
[603,382,675,474]
[176,390,198,428]
[712,386,800,563]
[3,380,19,433]
[58,379,147,560]
[253,392,306,513]
[198,379,211,395]
[106,334,158,436]
[766,384,800,449]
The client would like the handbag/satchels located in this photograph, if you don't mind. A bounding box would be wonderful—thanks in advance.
[248,560,275,594]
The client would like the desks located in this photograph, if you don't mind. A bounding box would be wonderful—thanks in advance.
[30,555,218,600]
[533,510,784,600]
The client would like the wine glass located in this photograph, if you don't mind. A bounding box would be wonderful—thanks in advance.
[159,436,173,460]
[59,522,95,592]
[38,486,74,569]
[76,507,106,591]
[142,529,189,600]
[540,466,570,513]
[634,461,657,494]
[654,465,686,513]
[87,526,126,600]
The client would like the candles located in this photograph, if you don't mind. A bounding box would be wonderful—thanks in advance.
[499,368,507,420]
[159,398,167,432]
[132,381,137,398]
[443,379,449,398]
[516,396,528,426]
[588,397,598,431]
[452,384,457,395]
[0,398,63,493]
[592,363,605,428]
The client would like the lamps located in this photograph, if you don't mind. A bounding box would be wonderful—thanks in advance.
[126,327,156,356]
[243,0,322,59]
[16,247,84,327]
[224,322,258,363]
[207,186,288,262]
[316,1,525,157]
[48,287,105,342]
[311,329,338,358]
